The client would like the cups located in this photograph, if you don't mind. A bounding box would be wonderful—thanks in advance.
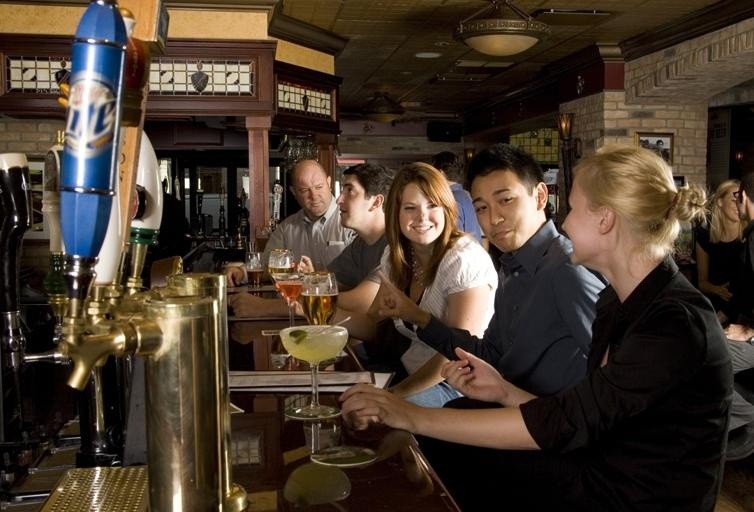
[245,252,265,288]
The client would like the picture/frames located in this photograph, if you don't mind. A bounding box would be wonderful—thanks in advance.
[633,130,675,169]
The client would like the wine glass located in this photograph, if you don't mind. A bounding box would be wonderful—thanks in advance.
[283,423,351,505]
[267,249,350,423]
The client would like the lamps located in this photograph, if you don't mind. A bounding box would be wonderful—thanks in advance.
[553,111,582,158]
[456,1,554,59]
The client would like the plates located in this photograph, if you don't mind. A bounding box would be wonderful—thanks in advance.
[310,446,377,468]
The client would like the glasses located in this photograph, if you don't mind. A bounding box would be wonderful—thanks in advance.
[733,191,741,198]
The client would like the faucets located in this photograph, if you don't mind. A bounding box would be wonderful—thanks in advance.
[0,153,164,391]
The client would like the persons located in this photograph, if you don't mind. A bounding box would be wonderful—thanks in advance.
[339,143,731,511]
[223,144,500,408]
[150,180,190,274]
[653,140,669,162]
[696,177,754,460]
[377,146,607,476]
